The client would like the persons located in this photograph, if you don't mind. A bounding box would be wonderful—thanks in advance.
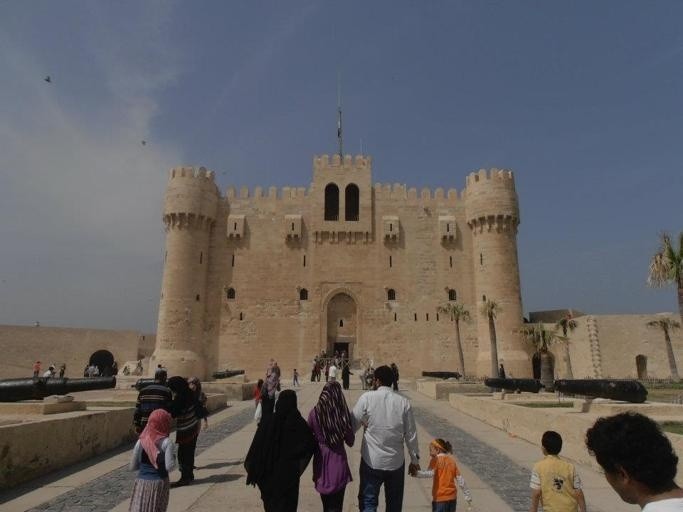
[529,431,586,512]
[243,390,316,512]
[350,366,422,512]
[254,348,400,424]
[411,439,474,512]
[498,364,505,378]
[32,359,209,511]
[307,381,355,512]
[585,411,683,512]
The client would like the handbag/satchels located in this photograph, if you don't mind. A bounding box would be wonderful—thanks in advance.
[261,383,267,399]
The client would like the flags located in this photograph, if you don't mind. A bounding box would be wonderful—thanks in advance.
[338,110,341,139]
